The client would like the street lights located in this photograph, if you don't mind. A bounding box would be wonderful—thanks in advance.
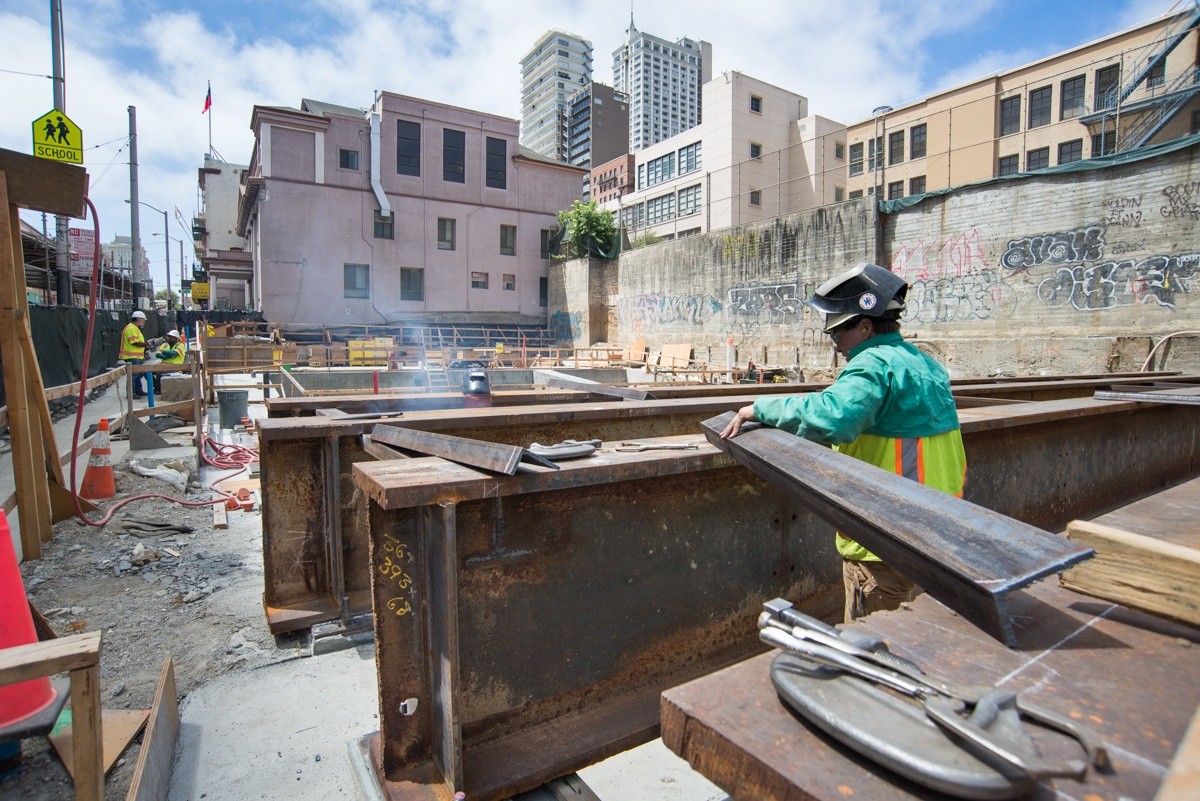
[125,199,172,314]
[151,233,186,312]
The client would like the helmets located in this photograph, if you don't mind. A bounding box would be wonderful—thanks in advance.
[804,260,909,334]
[132,311,147,320]
[167,330,181,338]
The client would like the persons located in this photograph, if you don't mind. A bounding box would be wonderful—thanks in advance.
[118,310,150,399]
[719,262,968,626]
[153,329,186,395]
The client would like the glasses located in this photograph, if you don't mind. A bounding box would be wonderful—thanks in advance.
[830,324,849,344]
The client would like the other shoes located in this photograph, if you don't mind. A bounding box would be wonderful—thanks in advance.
[133,394,140,399]
[137,391,148,396]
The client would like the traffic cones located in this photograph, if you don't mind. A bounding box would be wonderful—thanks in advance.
[0,506,74,741]
[78,418,130,502]
[180,326,188,343]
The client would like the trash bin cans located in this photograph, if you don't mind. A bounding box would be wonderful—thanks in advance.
[216,390,248,428]
